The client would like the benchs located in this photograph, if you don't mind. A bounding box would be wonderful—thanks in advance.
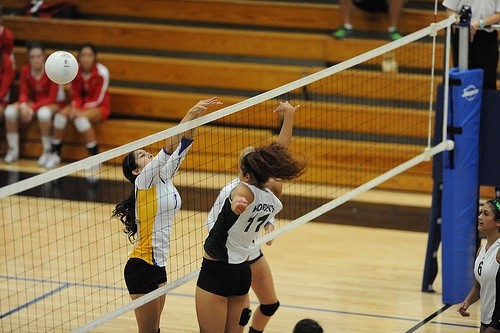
[0,0,499,194]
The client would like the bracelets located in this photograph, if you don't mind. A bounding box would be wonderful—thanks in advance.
[479,19,484,29]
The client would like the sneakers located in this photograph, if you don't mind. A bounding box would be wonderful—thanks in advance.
[47,154,60,168]
[5,148,20,163]
[38,152,52,166]
[334,25,356,38]
[387,27,402,40]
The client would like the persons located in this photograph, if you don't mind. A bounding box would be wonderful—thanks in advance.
[440,0,500,88]
[456,199,500,333]
[43,43,112,169]
[4,40,65,167]
[0,16,14,120]
[195,99,308,333]
[331,0,405,41]
[111,97,223,333]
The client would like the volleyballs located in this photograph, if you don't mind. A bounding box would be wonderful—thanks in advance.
[45,50,79,85]
